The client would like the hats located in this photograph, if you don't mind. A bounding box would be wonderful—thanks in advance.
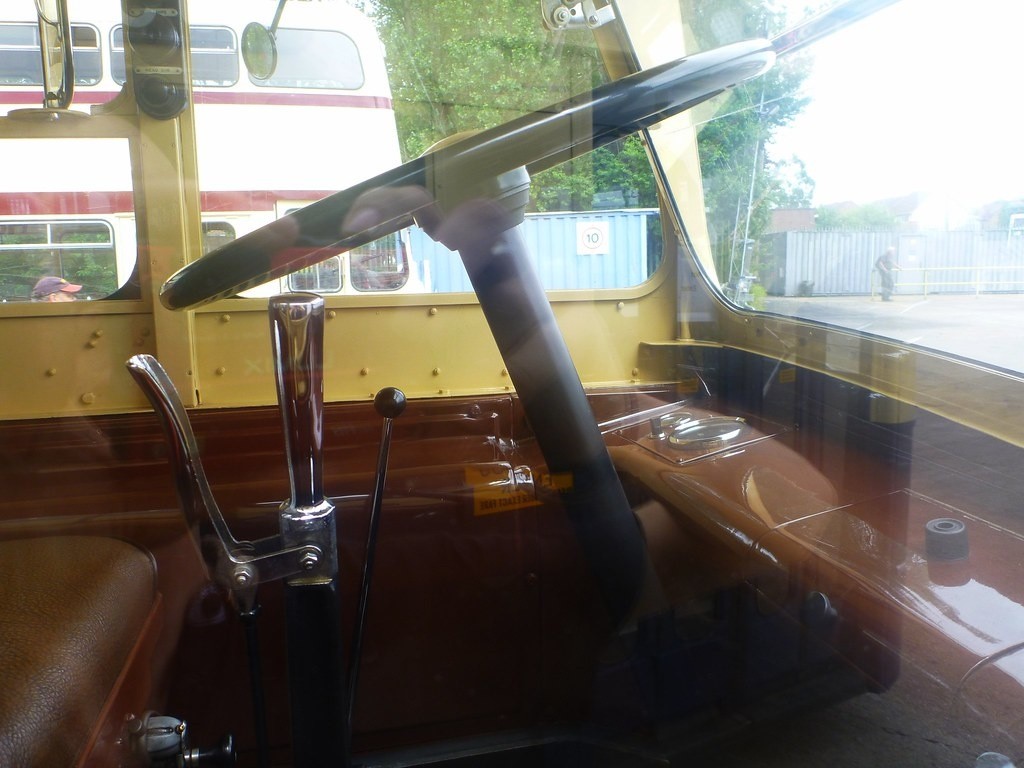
[30,276,82,298]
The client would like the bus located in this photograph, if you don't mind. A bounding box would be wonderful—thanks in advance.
[0,0,418,296]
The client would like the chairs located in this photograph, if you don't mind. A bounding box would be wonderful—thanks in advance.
[0,534,163,768]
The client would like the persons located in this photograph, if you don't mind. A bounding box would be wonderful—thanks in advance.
[874,246,903,303]
[30,274,84,302]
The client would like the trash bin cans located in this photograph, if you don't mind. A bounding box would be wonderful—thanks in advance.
[803,279,814,298]
